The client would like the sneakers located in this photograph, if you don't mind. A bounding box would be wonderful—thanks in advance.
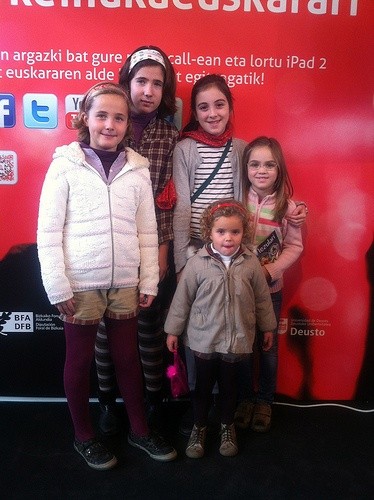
[128,432,177,462]
[233,398,273,433]
[185,424,207,459]
[73,436,117,470]
[219,422,238,457]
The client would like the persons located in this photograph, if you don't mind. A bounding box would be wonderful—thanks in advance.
[91,45,178,433]
[161,200,277,459]
[35,80,181,468]
[235,138,304,432]
[170,73,310,437]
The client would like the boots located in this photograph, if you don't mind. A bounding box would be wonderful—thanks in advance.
[147,389,164,430]
[98,386,118,434]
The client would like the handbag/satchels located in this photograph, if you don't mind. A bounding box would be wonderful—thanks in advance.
[170,348,189,397]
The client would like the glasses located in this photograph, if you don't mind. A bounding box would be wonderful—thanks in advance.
[247,161,277,170]
